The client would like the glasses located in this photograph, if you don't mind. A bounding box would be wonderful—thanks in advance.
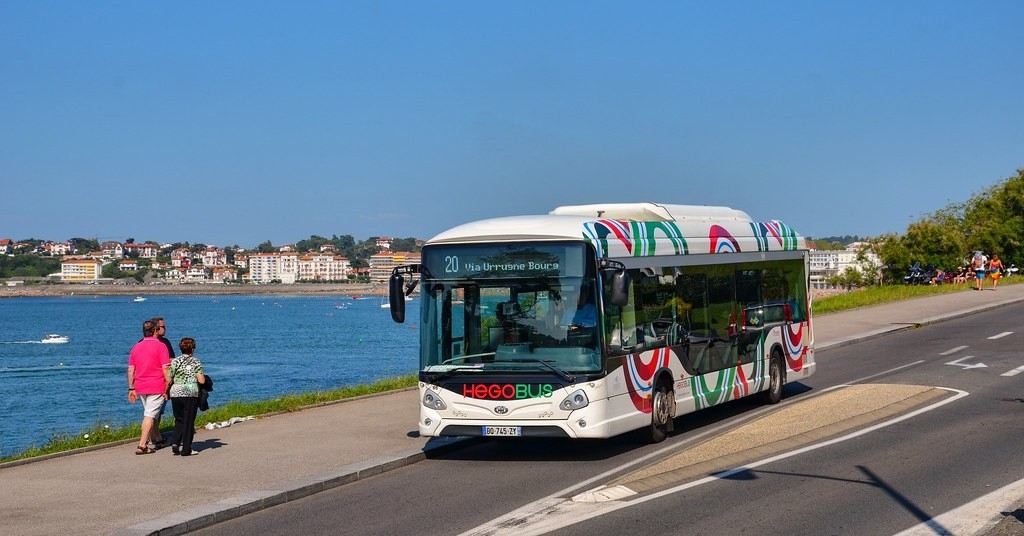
[158,324,165,328]
[157,328,160,330]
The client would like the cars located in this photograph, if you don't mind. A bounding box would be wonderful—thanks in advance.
[1006,264,1020,273]
[624,318,716,347]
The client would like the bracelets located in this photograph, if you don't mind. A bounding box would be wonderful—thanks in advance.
[129,387,135,391]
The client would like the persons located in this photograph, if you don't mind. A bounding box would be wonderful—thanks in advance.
[989,254,1006,290]
[930,262,992,287]
[970,251,987,291]
[169,337,206,456]
[558,288,597,330]
[127,320,171,454]
[141,318,175,444]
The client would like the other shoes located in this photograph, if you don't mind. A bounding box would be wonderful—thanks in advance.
[992,287,997,291]
[172,444,198,456]
[976,287,983,291]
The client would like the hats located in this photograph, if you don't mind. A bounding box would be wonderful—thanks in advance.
[957,266,962,269]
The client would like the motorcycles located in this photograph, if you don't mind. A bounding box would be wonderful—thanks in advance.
[904,262,936,286]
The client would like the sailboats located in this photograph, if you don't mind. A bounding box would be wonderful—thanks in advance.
[380,279,391,308]
[404,280,413,301]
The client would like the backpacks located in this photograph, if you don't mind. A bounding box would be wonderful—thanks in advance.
[974,256,982,268]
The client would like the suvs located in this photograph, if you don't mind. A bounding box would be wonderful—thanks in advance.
[728,300,803,337]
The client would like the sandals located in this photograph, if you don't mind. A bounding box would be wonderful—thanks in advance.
[156,436,168,444]
[135,445,156,455]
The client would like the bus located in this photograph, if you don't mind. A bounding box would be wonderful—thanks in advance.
[389,202,817,445]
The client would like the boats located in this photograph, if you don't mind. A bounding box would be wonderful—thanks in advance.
[450,298,465,305]
[41,334,70,344]
[478,302,489,309]
[134,296,147,302]
